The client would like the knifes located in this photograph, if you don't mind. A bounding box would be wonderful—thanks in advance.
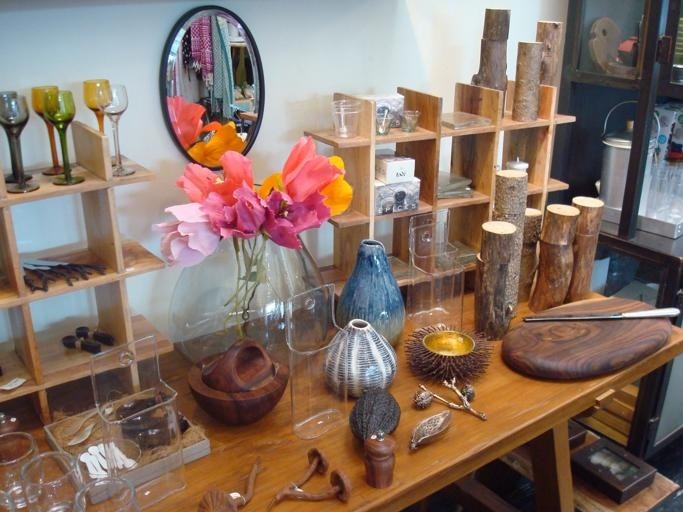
[521,308,681,326]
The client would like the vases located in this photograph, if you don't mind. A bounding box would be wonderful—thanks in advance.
[167,182,333,379]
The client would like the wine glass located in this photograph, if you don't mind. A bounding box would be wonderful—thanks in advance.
[0,80,137,195]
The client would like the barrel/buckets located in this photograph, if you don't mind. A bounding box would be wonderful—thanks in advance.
[601,101,661,213]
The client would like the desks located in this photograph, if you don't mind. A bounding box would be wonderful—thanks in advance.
[0,283,682,511]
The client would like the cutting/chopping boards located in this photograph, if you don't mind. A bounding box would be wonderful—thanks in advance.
[503,297,675,377]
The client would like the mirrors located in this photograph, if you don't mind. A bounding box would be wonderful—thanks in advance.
[160,4,264,173]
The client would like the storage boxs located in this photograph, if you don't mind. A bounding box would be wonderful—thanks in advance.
[373,178,421,215]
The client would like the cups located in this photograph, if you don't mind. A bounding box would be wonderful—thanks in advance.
[645,101,682,230]
[399,109,421,133]
[0,431,143,512]
[330,100,362,137]
[376,113,393,136]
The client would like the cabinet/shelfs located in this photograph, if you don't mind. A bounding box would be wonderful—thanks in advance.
[542,0,683,461]
[303,80,577,314]
[0,121,176,432]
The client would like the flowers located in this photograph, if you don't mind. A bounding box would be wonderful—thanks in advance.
[150,135,354,337]
[164,93,250,170]
